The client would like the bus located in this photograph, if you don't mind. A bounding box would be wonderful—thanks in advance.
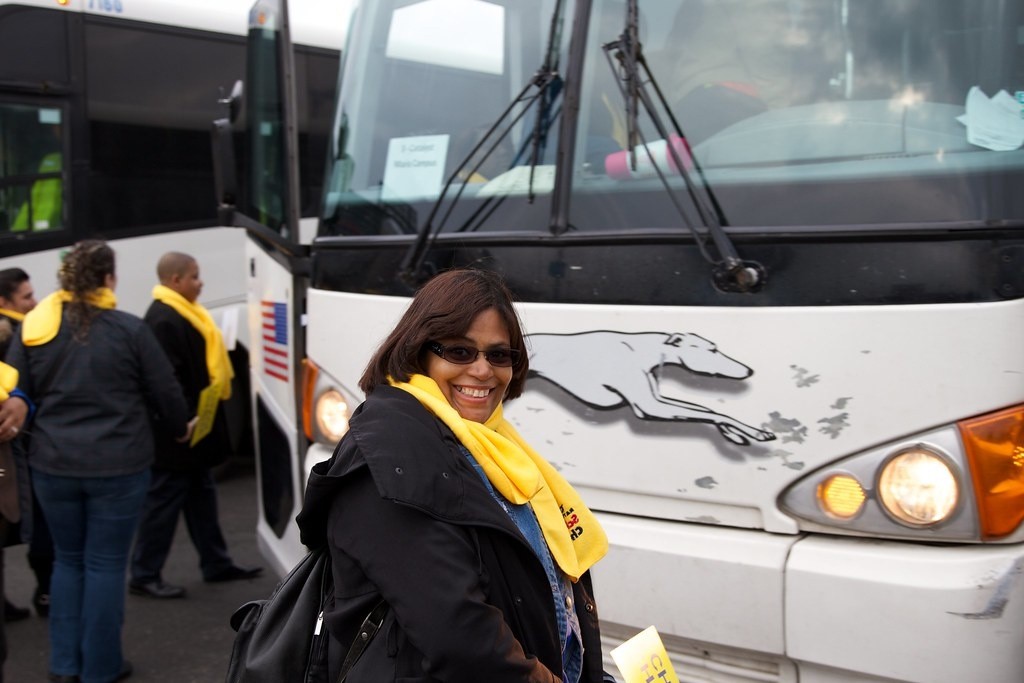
[0,0,513,481]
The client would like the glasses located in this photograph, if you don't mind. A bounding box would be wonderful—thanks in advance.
[423,340,522,367]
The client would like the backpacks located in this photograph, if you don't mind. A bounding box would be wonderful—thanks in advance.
[225,546,394,683]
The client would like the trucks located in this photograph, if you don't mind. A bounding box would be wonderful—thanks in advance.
[238,0,1024,682]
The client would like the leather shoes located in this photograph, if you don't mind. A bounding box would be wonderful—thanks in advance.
[129,578,187,599]
[203,562,263,584]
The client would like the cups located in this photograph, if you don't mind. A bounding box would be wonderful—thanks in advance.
[604,134,691,182]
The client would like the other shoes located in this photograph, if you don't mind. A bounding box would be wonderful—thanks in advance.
[0,594,32,623]
[30,590,51,617]
[47,673,80,683]
[0,629,8,665]
[110,662,133,683]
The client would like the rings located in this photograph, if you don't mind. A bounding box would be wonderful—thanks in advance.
[11,425,19,433]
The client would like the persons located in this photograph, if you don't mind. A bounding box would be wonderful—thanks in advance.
[4,240,193,683]
[127,252,264,602]
[0,268,38,683]
[297,270,612,683]
